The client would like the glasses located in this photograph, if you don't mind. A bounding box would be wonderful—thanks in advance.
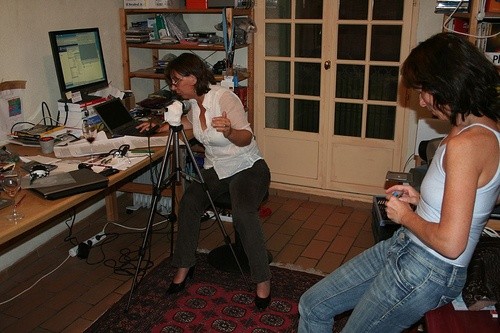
[172,72,187,87]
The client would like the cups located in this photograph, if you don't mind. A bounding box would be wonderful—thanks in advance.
[39,137,54,154]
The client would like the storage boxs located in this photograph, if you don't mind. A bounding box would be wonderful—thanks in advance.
[372,195,403,244]
[124,0,235,8]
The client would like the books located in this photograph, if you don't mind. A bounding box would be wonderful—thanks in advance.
[58,95,106,129]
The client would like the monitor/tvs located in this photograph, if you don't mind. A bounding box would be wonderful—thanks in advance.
[49,27,109,104]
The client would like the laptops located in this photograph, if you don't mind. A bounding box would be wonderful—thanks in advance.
[93,98,161,136]
[31,167,108,199]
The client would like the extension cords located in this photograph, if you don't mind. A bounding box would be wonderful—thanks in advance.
[204,210,233,222]
[69,232,106,258]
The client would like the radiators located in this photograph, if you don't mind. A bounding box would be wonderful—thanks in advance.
[131,161,173,212]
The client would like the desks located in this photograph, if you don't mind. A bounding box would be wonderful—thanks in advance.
[0,117,195,258]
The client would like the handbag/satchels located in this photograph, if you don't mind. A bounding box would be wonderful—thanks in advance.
[461,226,500,308]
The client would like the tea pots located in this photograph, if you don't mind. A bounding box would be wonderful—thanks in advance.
[211,61,227,75]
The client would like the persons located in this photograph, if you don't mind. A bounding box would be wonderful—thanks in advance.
[136,53,272,312]
[298,32,500,333]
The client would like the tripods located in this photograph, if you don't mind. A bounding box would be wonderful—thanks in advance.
[123,127,251,315]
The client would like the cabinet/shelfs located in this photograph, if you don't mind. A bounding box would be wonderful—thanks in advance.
[119,7,255,135]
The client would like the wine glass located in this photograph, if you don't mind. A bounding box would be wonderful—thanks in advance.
[81,123,98,163]
[1,170,24,221]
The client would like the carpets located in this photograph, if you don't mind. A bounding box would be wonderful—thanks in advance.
[81,246,355,333]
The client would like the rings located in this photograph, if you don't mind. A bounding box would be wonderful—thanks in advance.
[224,125,225,127]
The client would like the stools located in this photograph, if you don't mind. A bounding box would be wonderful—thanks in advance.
[206,190,273,270]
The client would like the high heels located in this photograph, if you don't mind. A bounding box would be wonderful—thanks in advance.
[255,288,272,310]
[166,265,196,294]
